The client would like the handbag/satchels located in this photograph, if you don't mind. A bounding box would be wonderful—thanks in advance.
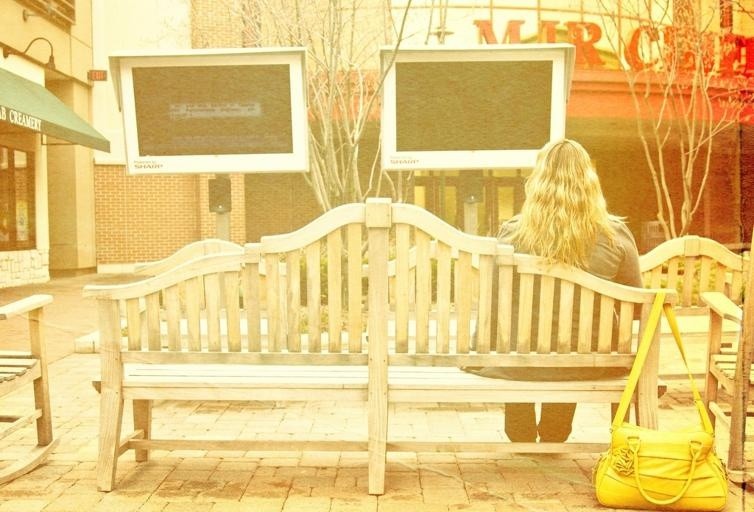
[592,290,729,510]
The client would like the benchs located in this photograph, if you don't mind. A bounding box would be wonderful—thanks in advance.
[94,239,289,355]
[76,196,679,497]
[614,233,754,335]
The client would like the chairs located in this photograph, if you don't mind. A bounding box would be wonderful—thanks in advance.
[699,247,754,481]
[0,294,63,485]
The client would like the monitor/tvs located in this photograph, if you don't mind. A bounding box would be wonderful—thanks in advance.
[379,44,578,172]
[110,48,314,176]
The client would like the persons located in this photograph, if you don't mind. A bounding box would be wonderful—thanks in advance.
[475,140,646,441]
